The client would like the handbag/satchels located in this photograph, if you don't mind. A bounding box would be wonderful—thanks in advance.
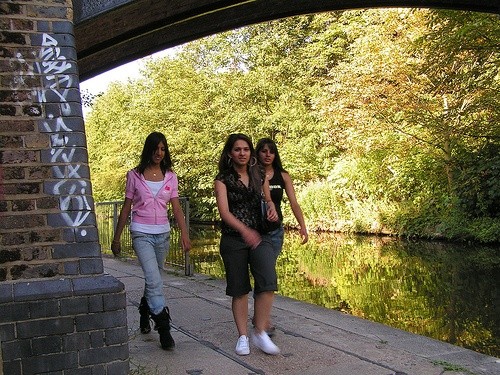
[257,196,280,233]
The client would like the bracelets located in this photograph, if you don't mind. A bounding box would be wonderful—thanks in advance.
[266,199,272,203]
[112,239,120,244]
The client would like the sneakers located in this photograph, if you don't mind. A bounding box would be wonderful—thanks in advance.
[235,335,250,355]
[252,329,280,354]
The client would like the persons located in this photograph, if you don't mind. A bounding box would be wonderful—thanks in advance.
[111,131,193,350]
[252,138,309,335]
[214,134,281,356]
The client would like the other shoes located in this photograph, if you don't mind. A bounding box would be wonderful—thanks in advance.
[251,317,275,335]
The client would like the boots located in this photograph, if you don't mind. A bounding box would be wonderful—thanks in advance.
[138,296,151,333]
[150,305,175,351]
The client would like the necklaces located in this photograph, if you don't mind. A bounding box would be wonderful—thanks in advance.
[146,168,162,176]
[265,169,274,178]
